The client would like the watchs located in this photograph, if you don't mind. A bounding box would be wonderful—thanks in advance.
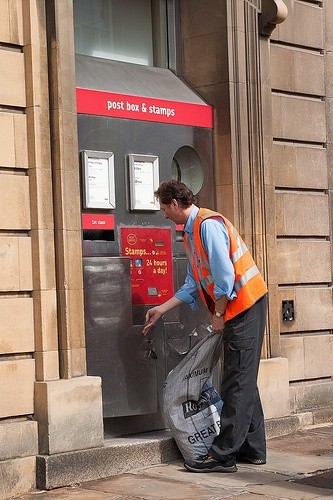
[214,311,223,318]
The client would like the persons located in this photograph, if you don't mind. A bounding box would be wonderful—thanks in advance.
[144,182,268,473]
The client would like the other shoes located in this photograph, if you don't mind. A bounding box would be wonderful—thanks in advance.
[238,453,267,465]
[184,452,237,473]
